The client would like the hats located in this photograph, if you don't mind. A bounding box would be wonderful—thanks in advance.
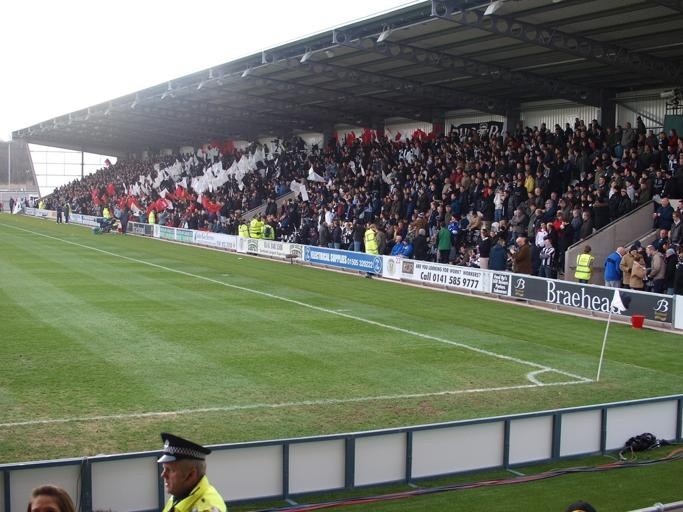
[156,432,211,464]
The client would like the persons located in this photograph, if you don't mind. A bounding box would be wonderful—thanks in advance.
[28,485,73,512]
[0,116,683,295]
[156,432,227,512]
